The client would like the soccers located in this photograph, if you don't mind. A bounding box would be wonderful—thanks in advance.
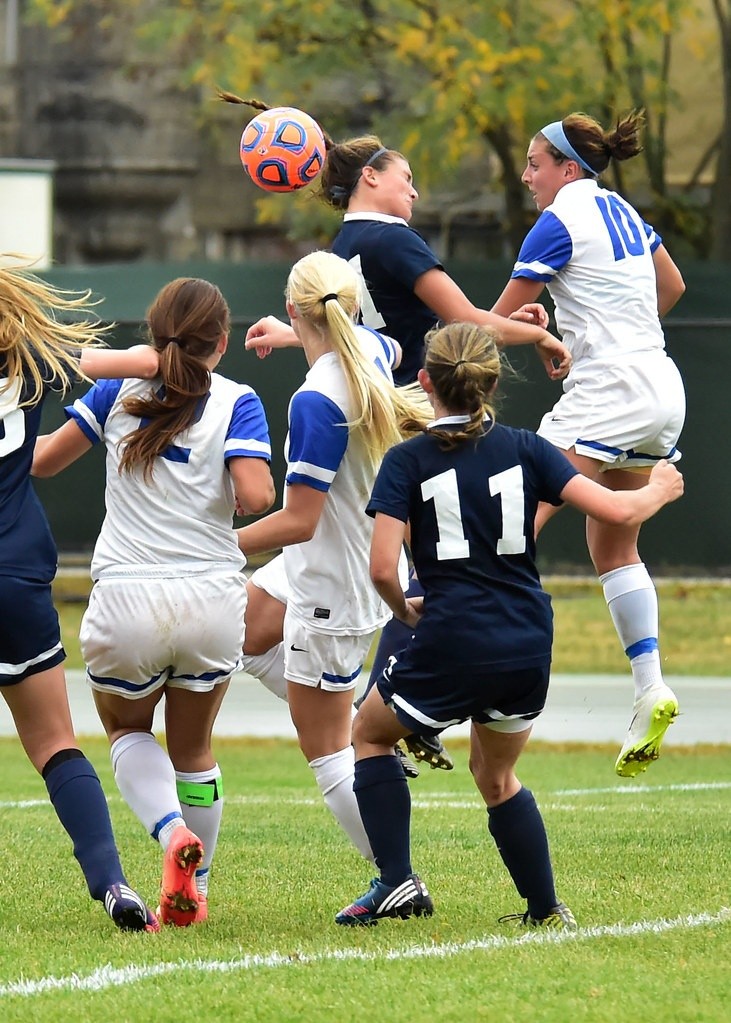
[237,106,327,193]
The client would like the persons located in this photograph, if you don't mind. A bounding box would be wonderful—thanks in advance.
[329,325,686,937]
[474,102,689,780]
[0,249,160,926]
[33,278,280,918]
[209,92,578,459]
[244,248,420,860]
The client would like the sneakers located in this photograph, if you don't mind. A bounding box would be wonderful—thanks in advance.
[333,874,434,925]
[153,893,208,924]
[512,899,576,932]
[157,824,203,927]
[397,730,455,771]
[103,883,161,934]
[613,682,679,777]
[393,744,420,778]
